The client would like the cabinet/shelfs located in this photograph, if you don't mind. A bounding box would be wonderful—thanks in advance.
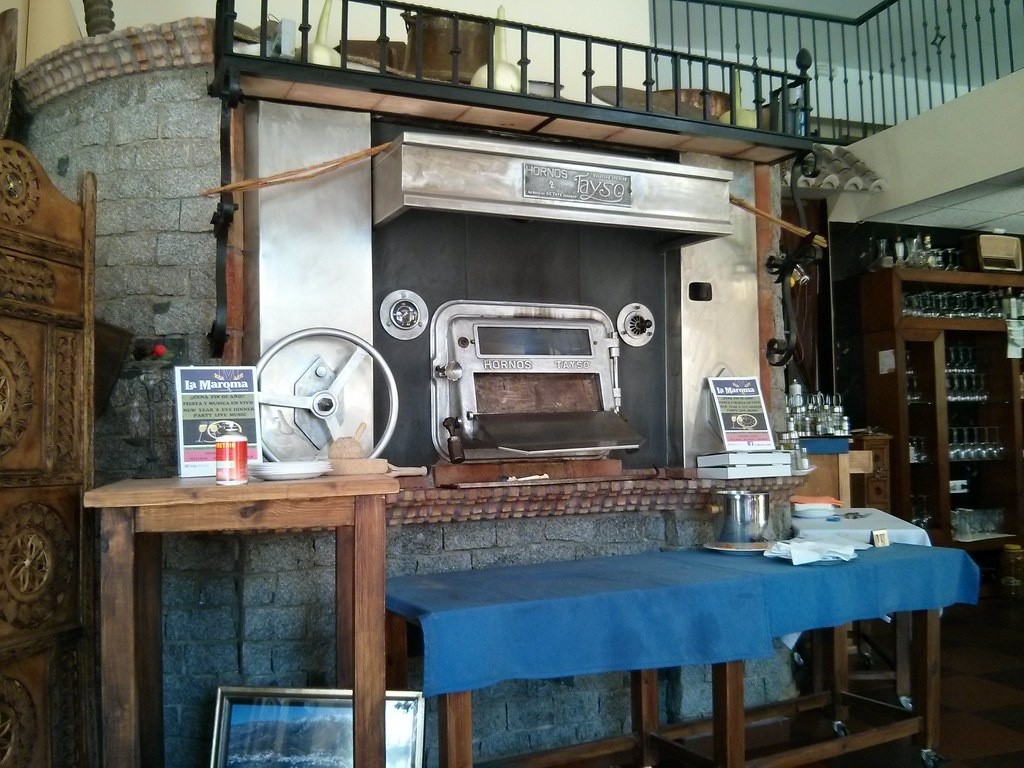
[850,266,1024,554]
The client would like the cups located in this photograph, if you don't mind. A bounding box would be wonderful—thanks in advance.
[711,491,771,548]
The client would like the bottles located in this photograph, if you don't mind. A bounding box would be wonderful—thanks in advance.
[216,428,249,485]
[784,440,809,470]
[786,378,850,438]
[904,350,935,396]
[858,237,904,267]
[1001,545,1024,603]
[1002,287,1024,320]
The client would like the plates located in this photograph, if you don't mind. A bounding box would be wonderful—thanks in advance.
[248,461,333,480]
[704,543,767,556]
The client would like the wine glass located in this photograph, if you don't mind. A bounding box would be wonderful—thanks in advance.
[910,495,934,529]
[950,507,1004,536]
[907,247,964,272]
[949,426,1006,459]
[908,435,928,462]
[946,344,990,400]
[903,291,1004,318]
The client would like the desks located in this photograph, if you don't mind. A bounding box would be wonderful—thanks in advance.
[788,507,934,700]
[386,543,980,768]
[82,472,401,768]
